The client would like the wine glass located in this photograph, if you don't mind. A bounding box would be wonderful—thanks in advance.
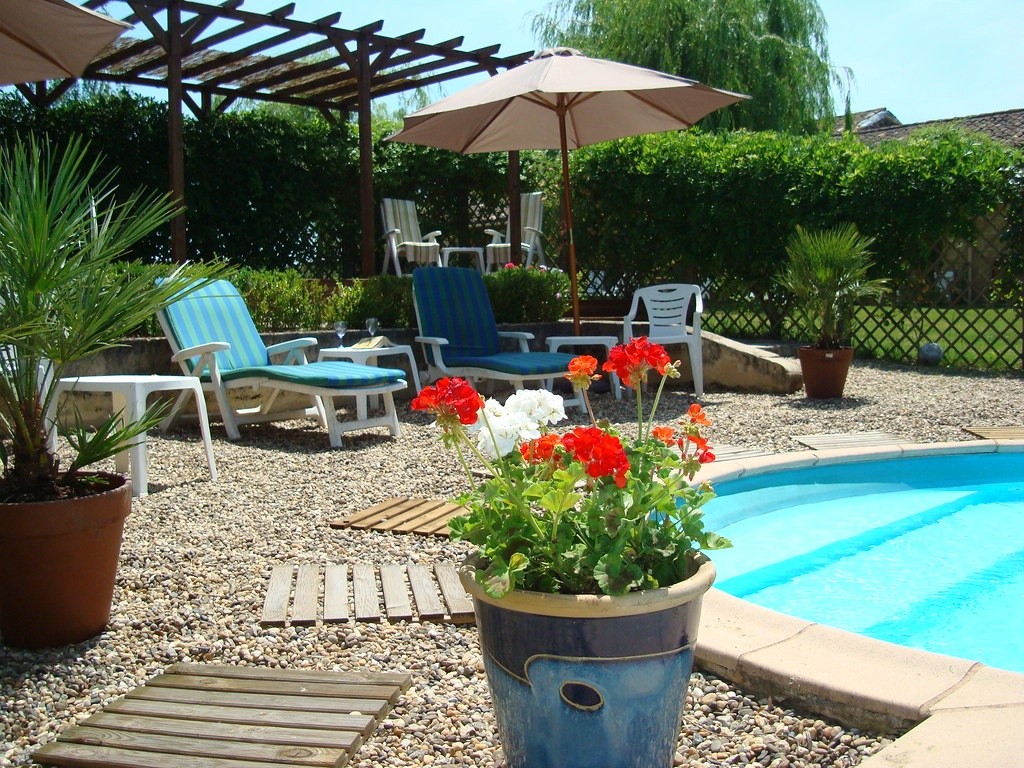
[334,321,347,349]
[365,318,378,336]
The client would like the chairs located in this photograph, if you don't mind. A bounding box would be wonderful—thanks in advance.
[617,283,704,395]
[152,277,404,448]
[0,293,53,400]
[380,199,441,275]
[411,266,593,416]
[482,191,546,274]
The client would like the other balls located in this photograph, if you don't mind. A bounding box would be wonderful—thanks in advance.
[919,343,942,366]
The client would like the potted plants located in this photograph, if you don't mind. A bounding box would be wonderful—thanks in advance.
[1,130,247,650]
[767,218,893,402]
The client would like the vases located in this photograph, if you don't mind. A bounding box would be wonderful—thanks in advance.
[452,546,718,768]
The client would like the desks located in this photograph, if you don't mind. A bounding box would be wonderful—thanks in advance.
[46,375,219,497]
[439,248,485,275]
[545,336,622,402]
[316,345,422,420]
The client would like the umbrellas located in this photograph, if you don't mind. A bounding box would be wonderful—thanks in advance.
[0,0,135,86]
[383,49,751,336]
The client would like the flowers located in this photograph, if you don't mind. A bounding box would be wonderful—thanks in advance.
[409,329,731,597]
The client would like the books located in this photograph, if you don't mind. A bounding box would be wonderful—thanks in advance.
[352,336,397,348]
